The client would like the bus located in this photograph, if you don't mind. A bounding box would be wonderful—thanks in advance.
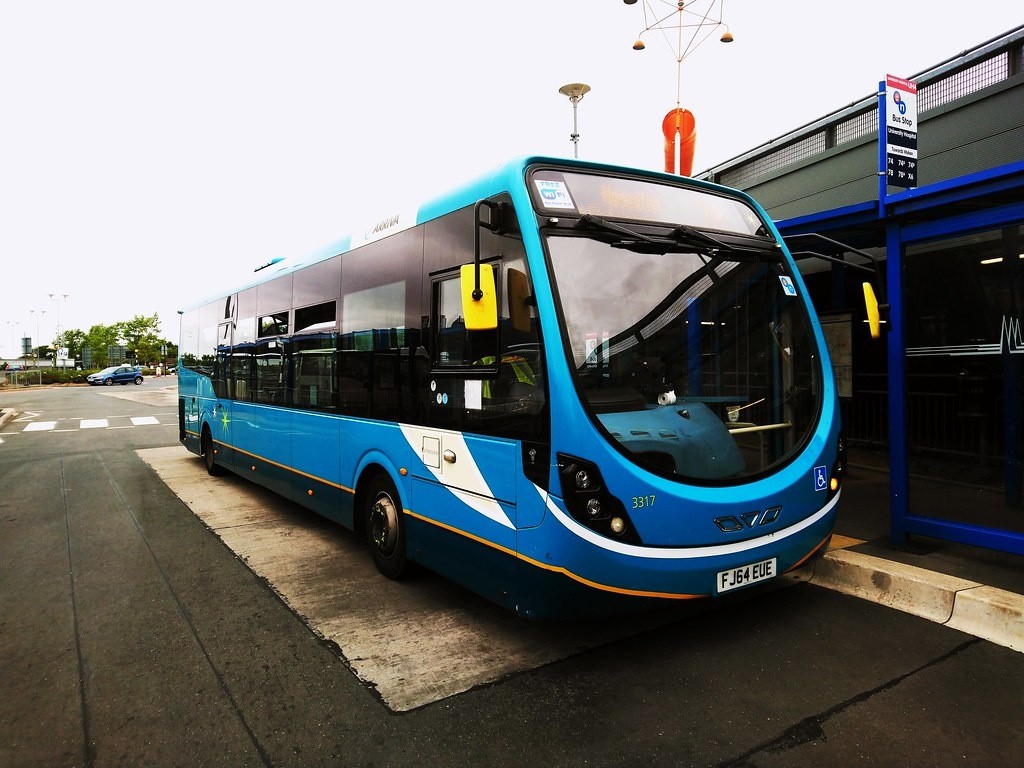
[212,349,385,412]
[176,154,891,627]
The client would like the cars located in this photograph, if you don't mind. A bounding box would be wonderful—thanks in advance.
[184,366,194,370]
[5,364,24,371]
[134,366,150,370]
[150,365,157,369]
[119,363,132,373]
[163,366,169,370]
[235,370,246,375]
[195,365,211,375]
[86,366,143,386]
[168,367,175,372]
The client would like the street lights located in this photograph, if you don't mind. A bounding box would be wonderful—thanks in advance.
[624,0,733,177]
[29,310,47,368]
[48,293,69,347]
[7,322,19,358]
[558,82,592,160]
